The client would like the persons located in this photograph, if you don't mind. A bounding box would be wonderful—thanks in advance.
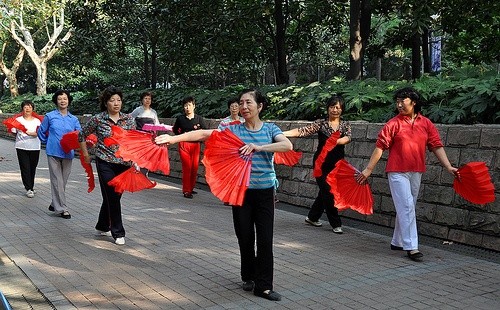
[356,88,461,258]
[281,95,352,233]
[155,89,293,300]
[173,97,208,198]
[12,101,41,198]
[218,97,245,205]
[77,87,137,244]
[128,91,160,177]
[37,90,83,219]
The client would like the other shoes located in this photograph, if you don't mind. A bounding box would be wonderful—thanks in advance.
[243,280,282,301]
[305,217,343,233]
[26,188,37,198]
[183,191,197,198]
[48,205,71,219]
[94,228,126,245]
[391,244,423,259]
